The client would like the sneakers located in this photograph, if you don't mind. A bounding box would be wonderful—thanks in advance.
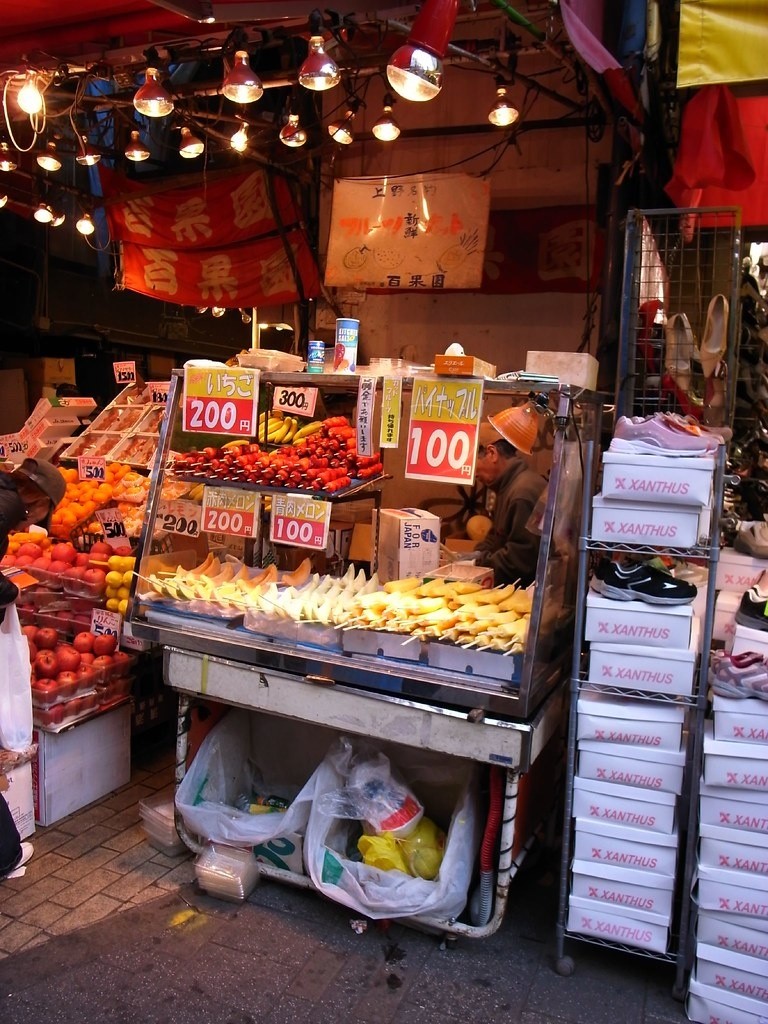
[735,585,768,631]
[590,557,698,605]
[734,521,768,559]
[708,649,759,686]
[713,657,768,701]
[609,411,733,456]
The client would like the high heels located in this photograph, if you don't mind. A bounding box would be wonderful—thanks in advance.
[700,294,729,378]
[661,373,700,421]
[703,376,725,427]
[665,313,691,393]
[639,300,659,369]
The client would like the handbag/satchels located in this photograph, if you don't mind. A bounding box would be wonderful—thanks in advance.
[0,603,34,752]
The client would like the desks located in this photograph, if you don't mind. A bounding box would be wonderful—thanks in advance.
[160,642,565,946]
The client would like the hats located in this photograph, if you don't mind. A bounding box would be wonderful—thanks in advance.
[478,423,505,447]
[17,457,67,533]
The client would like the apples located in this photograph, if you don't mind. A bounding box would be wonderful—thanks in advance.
[22,625,131,726]
[5,542,130,630]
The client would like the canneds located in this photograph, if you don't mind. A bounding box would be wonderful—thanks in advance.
[307,340,325,373]
[265,795,291,809]
[333,318,361,375]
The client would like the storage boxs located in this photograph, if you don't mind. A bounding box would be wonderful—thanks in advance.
[0,378,169,472]
[422,562,494,588]
[32,704,135,827]
[199,830,307,877]
[373,507,449,586]
[566,452,768,1024]
[244,611,422,663]
[427,617,574,684]
[525,350,599,393]
[0,760,36,841]
[0,541,228,730]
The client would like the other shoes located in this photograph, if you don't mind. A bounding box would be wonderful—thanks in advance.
[1,842,34,879]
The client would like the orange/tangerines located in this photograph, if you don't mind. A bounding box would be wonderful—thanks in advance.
[7,461,130,546]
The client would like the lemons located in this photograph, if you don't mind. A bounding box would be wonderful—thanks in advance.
[106,555,176,614]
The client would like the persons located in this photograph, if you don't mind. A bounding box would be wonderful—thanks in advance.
[457,422,551,589]
[0,457,68,878]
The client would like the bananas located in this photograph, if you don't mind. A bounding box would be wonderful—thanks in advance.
[219,408,325,455]
[188,482,205,500]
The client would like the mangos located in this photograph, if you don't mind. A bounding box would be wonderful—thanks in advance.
[73,473,183,540]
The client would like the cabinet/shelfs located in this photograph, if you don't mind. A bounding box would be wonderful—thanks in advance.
[552,439,730,1002]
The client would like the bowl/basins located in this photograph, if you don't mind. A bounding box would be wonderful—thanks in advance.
[235,348,306,373]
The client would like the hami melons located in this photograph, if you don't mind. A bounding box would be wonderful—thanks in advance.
[149,552,561,653]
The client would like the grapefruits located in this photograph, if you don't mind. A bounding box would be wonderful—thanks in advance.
[394,816,443,880]
